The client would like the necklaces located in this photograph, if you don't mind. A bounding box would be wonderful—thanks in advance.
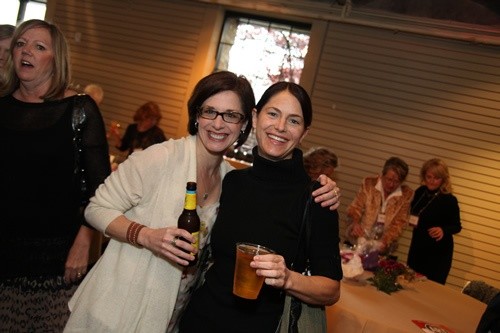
[199,178,215,199]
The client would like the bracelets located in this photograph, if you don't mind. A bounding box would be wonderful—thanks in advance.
[126,222,146,248]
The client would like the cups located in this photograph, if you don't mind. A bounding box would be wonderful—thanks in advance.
[233,242,275,300]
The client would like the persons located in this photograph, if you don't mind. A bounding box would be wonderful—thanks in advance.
[303,147,338,182]
[0,24,17,85]
[346,157,414,259]
[172,81,345,333]
[0,20,112,333]
[117,101,167,157]
[62,71,342,333]
[85,84,103,104]
[406,158,463,285]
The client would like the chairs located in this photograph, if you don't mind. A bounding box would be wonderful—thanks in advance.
[461,279,500,304]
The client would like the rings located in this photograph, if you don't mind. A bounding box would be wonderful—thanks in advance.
[334,190,337,195]
[77,273,81,275]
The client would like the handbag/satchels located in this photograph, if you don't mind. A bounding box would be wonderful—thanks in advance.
[275,265,328,333]
[87,227,103,264]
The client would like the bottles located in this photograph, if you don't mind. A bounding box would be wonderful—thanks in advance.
[177,181,201,267]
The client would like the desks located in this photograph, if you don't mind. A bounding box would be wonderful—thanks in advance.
[326,274,488,333]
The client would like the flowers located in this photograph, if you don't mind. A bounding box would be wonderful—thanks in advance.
[365,255,415,296]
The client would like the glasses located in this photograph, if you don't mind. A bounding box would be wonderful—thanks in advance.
[200,106,245,123]
